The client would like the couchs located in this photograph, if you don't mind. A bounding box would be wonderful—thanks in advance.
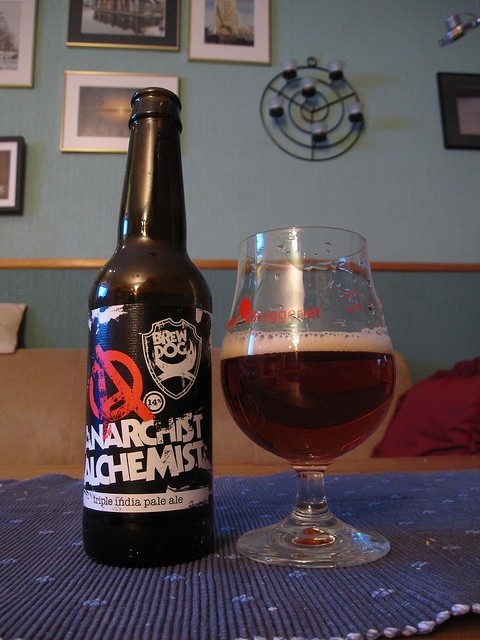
[1,349,479,479]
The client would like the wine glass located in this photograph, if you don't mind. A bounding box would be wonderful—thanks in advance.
[219,225,397,570]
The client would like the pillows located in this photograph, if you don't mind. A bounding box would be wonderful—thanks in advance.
[373,364,479,462]
[0,300,26,355]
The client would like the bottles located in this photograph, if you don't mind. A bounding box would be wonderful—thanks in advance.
[82,87,216,567]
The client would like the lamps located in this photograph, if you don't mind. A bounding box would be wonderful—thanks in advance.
[258,54,363,163]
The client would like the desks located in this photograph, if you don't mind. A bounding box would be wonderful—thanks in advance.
[0,461,479,639]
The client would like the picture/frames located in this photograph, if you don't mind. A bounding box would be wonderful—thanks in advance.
[65,0,181,52]
[60,69,181,154]
[0,0,39,88]
[437,71,479,150]
[187,0,273,68]
[0,134,26,214]
